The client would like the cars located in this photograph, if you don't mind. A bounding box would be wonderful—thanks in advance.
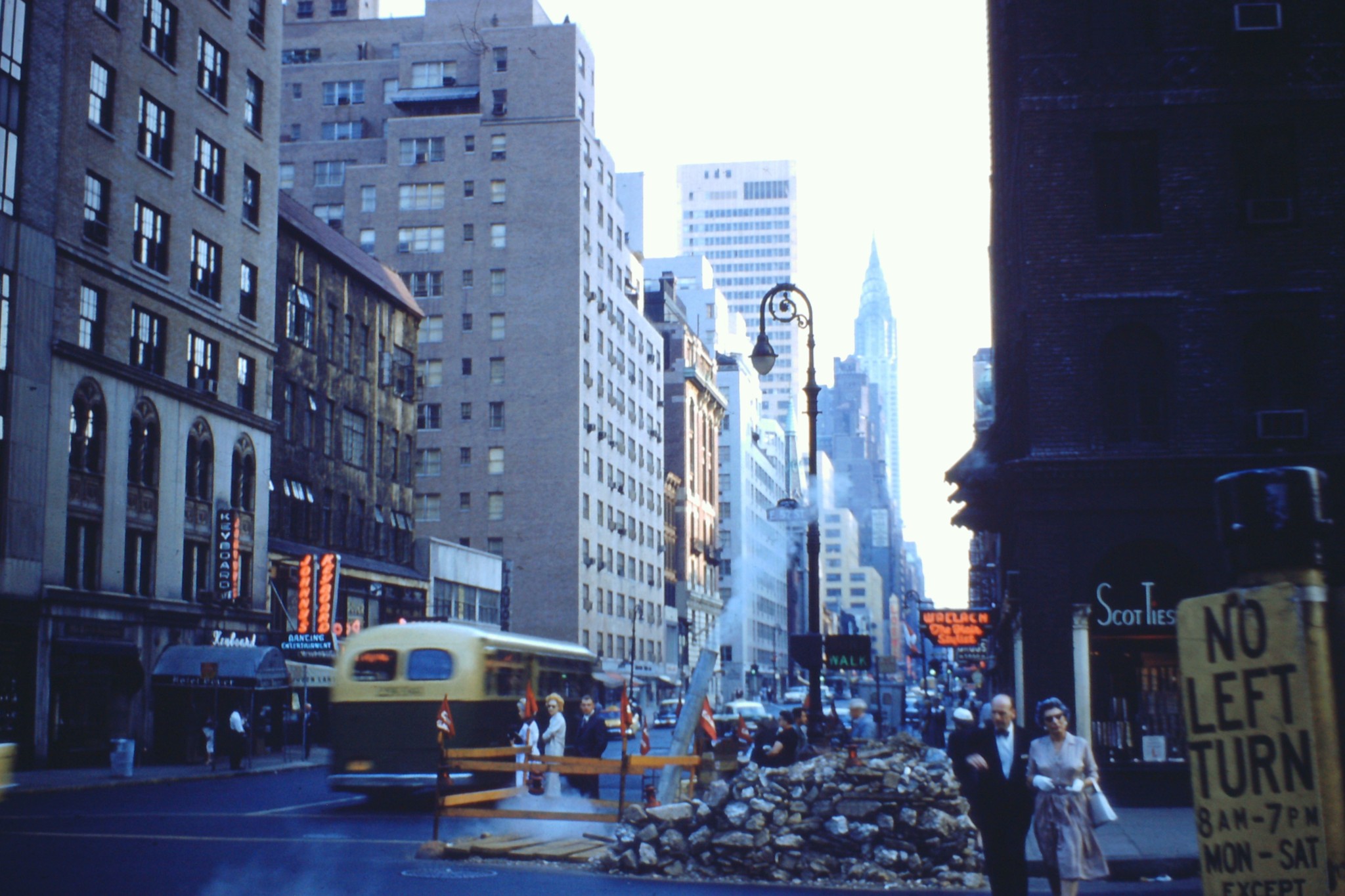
[821,706,856,738]
[653,699,687,726]
[710,702,775,734]
[784,675,873,704]
[602,706,637,740]
[903,684,947,727]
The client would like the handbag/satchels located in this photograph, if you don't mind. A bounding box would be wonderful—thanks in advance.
[1083,775,1117,825]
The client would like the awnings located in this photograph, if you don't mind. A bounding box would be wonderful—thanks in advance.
[151,644,290,758]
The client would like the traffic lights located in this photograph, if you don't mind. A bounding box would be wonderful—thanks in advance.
[928,657,943,677]
[947,665,954,674]
[750,663,757,676]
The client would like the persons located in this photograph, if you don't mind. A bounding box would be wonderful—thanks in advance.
[957,695,1034,896]
[573,696,608,800]
[759,682,777,704]
[735,685,743,699]
[1026,697,1110,896]
[543,692,566,797]
[229,704,246,770]
[922,674,993,749]
[202,717,216,769]
[511,698,542,788]
[791,707,808,752]
[764,711,801,768]
[850,698,878,745]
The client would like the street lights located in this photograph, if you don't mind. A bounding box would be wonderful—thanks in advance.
[903,588,929,694]
[745,281,826,752]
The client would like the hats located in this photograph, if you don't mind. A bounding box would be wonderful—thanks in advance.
[951,707,978,729]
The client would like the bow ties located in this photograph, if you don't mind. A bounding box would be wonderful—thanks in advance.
[994,727,1009,738]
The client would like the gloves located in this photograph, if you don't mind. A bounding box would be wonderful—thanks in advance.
[1032,774,1054,792]
[1065,779,1085,793]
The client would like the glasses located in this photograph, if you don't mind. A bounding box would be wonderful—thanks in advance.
[548,705,559,708]
[1043,712,1064,722]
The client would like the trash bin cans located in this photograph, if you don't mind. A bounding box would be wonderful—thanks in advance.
[0,742,18,788]
[109,738,135,777]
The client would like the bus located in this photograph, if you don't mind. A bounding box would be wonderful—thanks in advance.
[323,620,607,804]
